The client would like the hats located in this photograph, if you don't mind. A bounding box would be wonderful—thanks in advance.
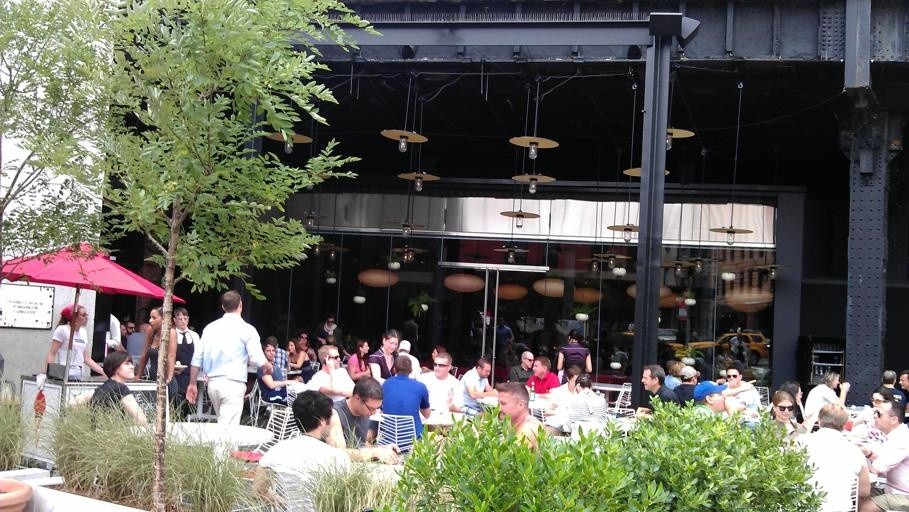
[693,381,727,401]
[680,366,700,378]
[399,340,411,352]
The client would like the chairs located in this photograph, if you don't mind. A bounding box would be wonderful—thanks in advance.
[1,356,908,512]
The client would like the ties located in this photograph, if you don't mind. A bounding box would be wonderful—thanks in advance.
[181,333,188,348]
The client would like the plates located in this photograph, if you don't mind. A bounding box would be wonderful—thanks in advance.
[175,365,188,369]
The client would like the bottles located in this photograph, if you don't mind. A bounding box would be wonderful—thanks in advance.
[529,382,535,401]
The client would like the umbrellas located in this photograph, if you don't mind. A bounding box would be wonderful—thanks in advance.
[0,241,187,383]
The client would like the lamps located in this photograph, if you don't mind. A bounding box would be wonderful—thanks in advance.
[266,237,778,311]
[606,72,695,243]
[271,99,313,152]
[379,72,441,238]
[707,82,753,245]
[301,186,323,229]
[500,71,558,236]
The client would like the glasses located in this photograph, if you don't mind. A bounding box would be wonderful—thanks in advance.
[329,355,340,360]
[432,363,449,368]
[726,375,739,378]
[873,409,882,419]
[363,399,384,412]
[776,405,793,412]
[870,396,882,405]
